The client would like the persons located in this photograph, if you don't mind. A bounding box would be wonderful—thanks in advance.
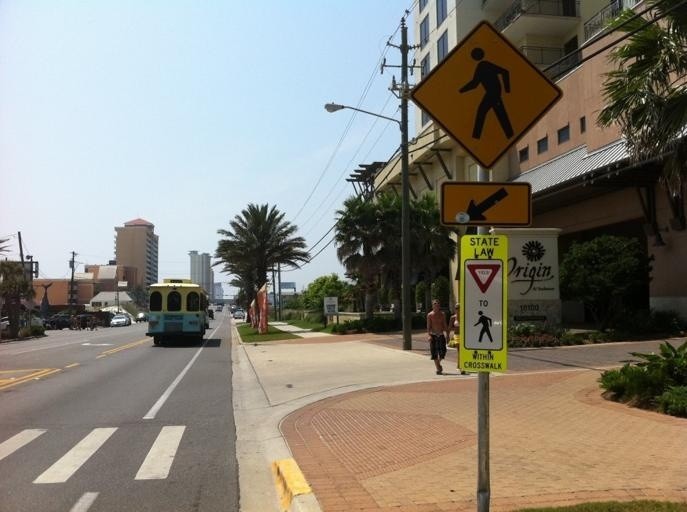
[427,298,450,376]
[446,303,465,375]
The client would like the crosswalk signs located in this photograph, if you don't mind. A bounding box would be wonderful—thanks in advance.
[459,235,508,373]
[411,20,563,169]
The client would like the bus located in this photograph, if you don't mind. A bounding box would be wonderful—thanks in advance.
[146,278,209,347]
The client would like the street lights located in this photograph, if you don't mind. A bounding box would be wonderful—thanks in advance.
[324,101,412,350]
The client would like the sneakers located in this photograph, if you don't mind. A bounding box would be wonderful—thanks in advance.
[434,365,469,376]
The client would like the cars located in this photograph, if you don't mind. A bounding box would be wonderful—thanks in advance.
[0,316,10,329]
[208,309,214,319]
[230,305,245,319]
[136,313,146,323]
[110,314,131,327]
[216,305,222,312]
